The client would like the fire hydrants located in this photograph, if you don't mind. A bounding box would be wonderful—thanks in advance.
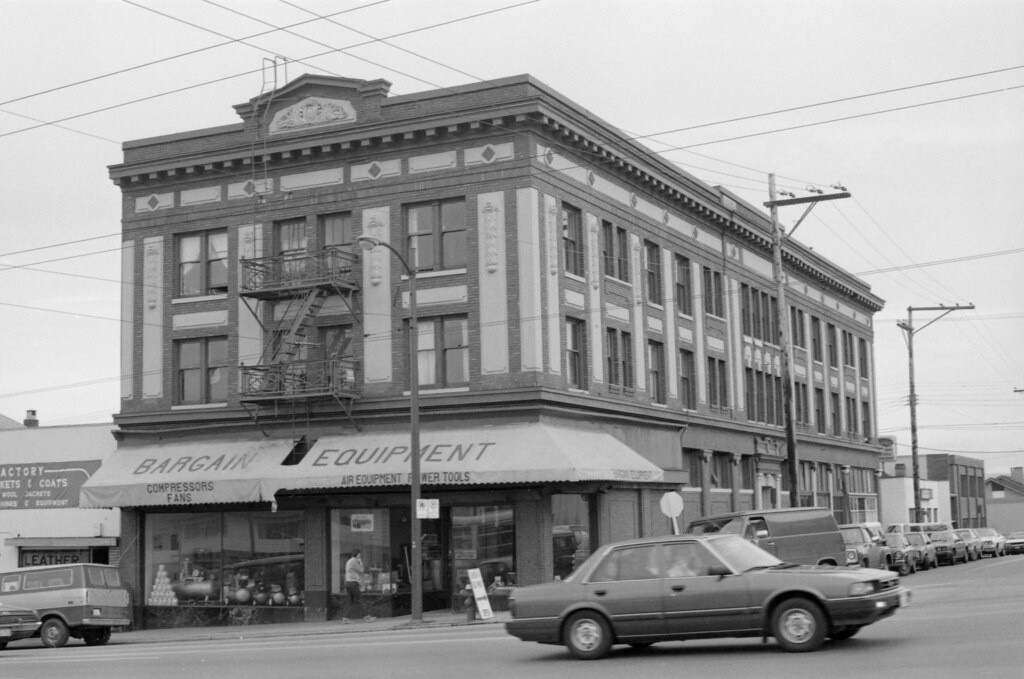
[460,584,477,620]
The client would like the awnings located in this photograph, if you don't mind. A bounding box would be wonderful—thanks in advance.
[78,423,671,512]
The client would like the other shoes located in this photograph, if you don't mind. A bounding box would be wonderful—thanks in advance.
[368,617,376,623]
[343,618,349,623]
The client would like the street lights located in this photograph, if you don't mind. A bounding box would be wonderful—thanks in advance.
[357,237,433,619]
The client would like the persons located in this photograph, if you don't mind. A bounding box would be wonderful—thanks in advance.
[341,549,377,624]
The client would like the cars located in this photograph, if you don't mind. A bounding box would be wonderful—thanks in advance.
[506,534,911,660]
[506,507,1024,659]
[0,562,134,648]
